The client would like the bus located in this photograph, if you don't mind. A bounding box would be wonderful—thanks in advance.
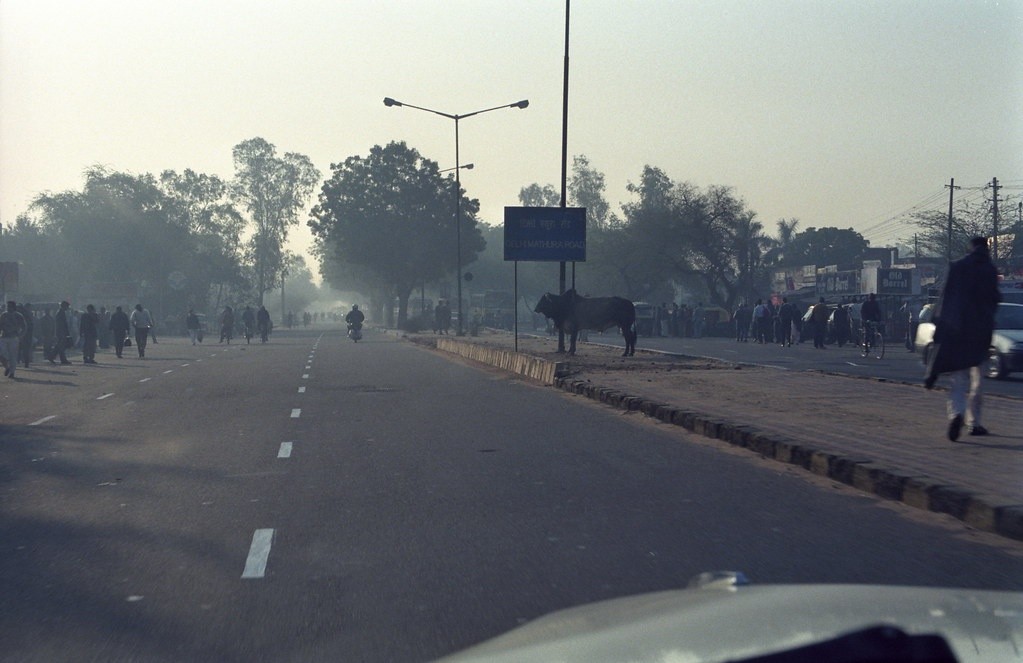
[468,290,515,330]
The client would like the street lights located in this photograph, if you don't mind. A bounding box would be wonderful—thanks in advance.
[383,96,530,337]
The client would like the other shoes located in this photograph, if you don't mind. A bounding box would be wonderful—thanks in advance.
[968,425,988,435]
[4,369,9,376]
[9,374,15,379]
[948,414,961,440]
[62,359,72,365]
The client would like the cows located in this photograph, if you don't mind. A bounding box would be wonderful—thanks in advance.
[542,289,638,356]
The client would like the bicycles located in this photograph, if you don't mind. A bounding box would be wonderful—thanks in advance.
[855,321,885,360]
[223,329,232,346]
[245,322,252,344]
[261,324,269,344]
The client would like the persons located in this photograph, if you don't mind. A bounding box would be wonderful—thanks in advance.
[932,237,1002,442]
[777,298,794,347]
[219,305,234,343]
[650,301,706,338]
[899,298,935,365]
[433,299,452,335]
[860,292,882,353]
[242,306,255,338]
[256,306,270,341]
[733,298,802,345]
[130,304,153,357]
[812,297,830,349]
[346,304,364,335]
[187,309,202,346]
[110,306,130,358]
[833,296,862,348]
[0,301,110,368]
[0,301,26,379]
[287,311,325,328]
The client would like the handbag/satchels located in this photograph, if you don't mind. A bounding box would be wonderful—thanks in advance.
[124,337,132,346]
[197,330,203,342]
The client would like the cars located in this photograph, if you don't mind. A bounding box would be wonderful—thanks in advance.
[914,303,1023,383]
[188,314,208,331]
[27,300,77,351]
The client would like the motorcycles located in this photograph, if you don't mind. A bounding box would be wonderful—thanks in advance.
[346,320,364,343]
[702,308,731,338]
[618,301,656,338]
